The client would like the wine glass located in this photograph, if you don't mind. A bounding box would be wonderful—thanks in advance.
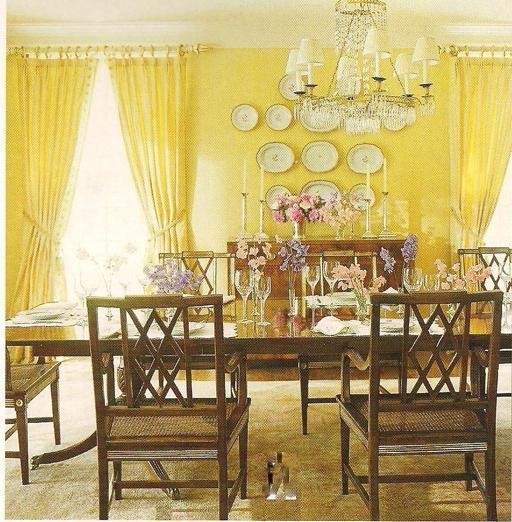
[399,265,441,322]
[71,268,102,327]
[496,260,511,302]
[233,268,272,327]
[303,259,339,313]
[498,300,510,335]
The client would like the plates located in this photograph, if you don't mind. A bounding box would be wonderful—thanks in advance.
[160,321,206,336]
[373,318,413,332]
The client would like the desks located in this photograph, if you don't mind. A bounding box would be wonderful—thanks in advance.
[223,237,414,368]
[6,294,509,501]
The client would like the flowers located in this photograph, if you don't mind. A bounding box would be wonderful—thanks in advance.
[231,188,421,290]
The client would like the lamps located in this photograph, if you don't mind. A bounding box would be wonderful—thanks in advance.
[283,0,442,138]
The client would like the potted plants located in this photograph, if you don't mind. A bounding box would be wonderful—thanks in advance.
[241,155,388,198]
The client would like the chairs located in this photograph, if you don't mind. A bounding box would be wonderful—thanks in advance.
[328,291,507,522]
[281,245,412,439]
[451,245,510,425]
[150,249,243,430]
[82,292,250,518]
[6,343,68,487]
